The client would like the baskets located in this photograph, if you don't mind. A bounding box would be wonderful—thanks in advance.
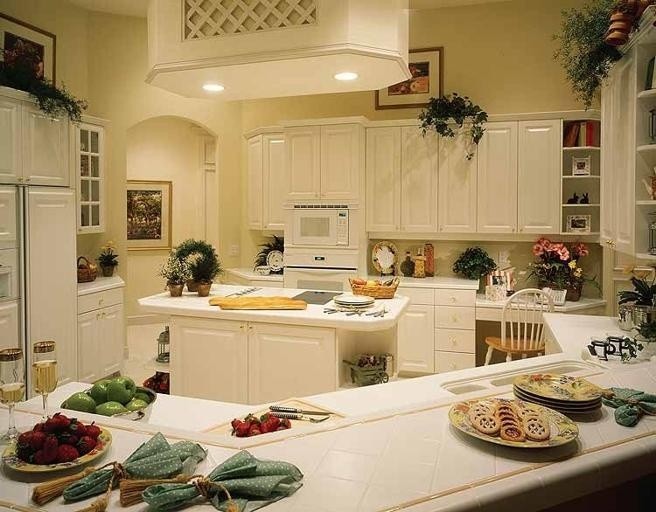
[348,277,400,298]
[77,256,97,283]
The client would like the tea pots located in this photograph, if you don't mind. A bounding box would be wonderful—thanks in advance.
[641,166,656,200]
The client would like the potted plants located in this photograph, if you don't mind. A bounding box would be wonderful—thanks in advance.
[617,276,655,331]
[174,238,226,297]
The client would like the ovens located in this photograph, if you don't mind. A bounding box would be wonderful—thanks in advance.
[283,268,359,293]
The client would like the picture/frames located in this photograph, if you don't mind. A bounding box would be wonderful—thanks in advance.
[126,179,173,250]
[0,12,56,91]
[374,46,443,109]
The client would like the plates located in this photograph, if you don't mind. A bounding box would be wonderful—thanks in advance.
[3,424,112,471]
[451,397,578,455]
[335,294,374,308]
[371,240,400,274]
[511,374,604,414]
[266,250,284,272]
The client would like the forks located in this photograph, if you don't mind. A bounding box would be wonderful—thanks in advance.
[271,411,331,424]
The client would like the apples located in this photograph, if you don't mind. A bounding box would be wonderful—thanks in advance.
[65,375,152,416]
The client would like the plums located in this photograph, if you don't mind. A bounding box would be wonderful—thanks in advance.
[78,262,98,269]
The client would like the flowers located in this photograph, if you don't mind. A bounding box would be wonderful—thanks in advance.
[533,239,587,283]
[94,239,119,263]
[156,248,205,282]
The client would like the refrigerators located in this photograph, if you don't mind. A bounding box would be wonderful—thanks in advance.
[0,183,80,400]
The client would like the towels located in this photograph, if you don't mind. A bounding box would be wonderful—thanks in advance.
[64,431,208,499]
[139,450,304,511]
[603,385,656,427]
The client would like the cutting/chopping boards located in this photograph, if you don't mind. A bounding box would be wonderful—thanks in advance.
[198,396,342,437]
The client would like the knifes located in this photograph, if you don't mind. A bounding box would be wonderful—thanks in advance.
[270,404,334,415]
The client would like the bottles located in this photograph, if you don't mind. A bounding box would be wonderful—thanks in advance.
[414,248,426,278]
[424,243,434,276]
[399,251,414,276]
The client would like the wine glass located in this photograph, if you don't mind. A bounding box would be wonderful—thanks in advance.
[30,339,59,423]
[0,346,27,444]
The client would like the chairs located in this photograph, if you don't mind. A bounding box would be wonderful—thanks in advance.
[483,288,553,363]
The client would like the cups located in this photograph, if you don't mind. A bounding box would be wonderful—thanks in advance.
[256,266,271,275]
[589,337,631,360]
[619,303,635,333]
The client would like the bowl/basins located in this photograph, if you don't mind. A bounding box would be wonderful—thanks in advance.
[60,387,156,425]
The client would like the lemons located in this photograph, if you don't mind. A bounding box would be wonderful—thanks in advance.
[365,280,376,286]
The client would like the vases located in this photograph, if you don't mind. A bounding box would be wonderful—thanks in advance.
[537,278,584,299]
[166,282,184,297]
[99,263,115,275]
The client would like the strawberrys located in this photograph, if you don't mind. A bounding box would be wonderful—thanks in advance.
[14,412,101,465]
[231,412,292,438]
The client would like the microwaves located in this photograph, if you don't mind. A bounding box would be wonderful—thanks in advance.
[291,210,349,246]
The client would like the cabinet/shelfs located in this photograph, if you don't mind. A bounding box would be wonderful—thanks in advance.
[634,41,656,259]
[26,185,76,399]
[365,123,438,233]
[243,131,285,230]
[439,121,558,234]
[70,117,107,234]
[600,47,635,260]
[76,275,125,383]
[564,120,602,240]
[2,90,69,184]
[169,315,400,405]
[286,122,360,201]
[0,183,25,390]
[392,277,478,370]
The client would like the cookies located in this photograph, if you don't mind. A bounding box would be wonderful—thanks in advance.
[469,398,551,443]
